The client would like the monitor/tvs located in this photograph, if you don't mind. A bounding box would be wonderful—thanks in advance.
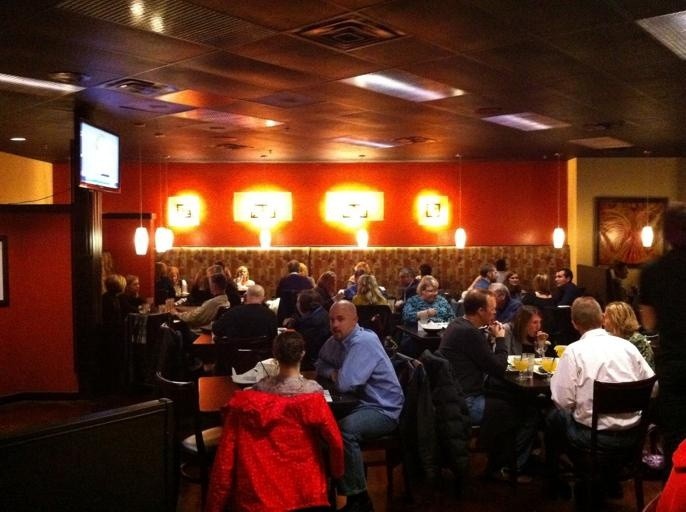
[73,116,122,194]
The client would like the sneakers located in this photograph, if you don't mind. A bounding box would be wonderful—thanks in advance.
[338,497,374,511]
[493,467,533,483]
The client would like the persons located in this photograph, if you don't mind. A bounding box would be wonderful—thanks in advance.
[543,296,659,503]
[310,299,405,512]
[246,331,324,400]
[94,254,389,376]
[400,259,656,377]
[438,287,519,484]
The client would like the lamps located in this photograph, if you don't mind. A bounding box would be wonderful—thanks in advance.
[132,138,150,255]
[154,157,173,254]
[455,153,466,250]
[641,149,654,249]
[552,153,566,249]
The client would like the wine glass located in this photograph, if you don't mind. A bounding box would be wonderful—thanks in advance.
[542,359,557,382]
[514,357,528,380]
[535,341,547,358]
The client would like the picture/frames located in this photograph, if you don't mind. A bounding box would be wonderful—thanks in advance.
[593,196,671,270]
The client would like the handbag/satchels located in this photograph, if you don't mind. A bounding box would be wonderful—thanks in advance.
[642,423,667,470]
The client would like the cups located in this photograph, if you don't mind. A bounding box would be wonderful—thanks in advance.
[522,353,536,379]
[166,298,174,312]
[144,303,150,312]
